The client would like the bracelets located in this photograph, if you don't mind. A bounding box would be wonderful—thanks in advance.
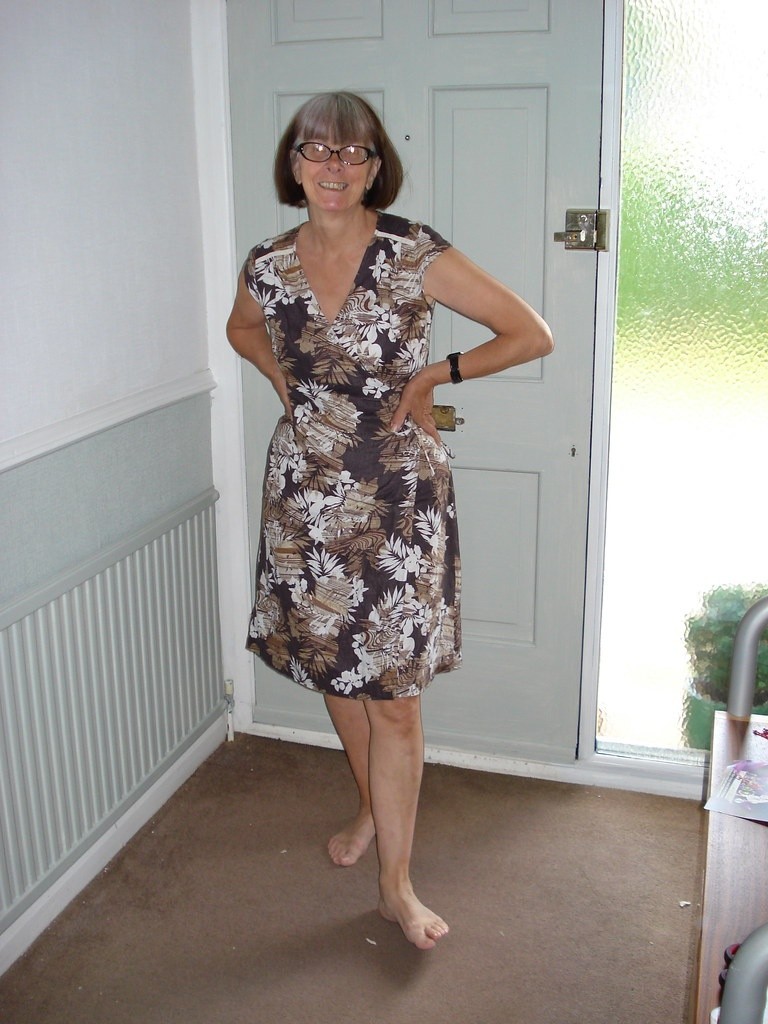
[446,351,465,384]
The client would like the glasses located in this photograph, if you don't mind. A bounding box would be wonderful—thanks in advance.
[296,142,376,166]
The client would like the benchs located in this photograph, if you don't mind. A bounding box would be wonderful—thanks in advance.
[695,595,768,1024]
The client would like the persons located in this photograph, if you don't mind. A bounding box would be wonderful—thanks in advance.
[226,92,556,952]
